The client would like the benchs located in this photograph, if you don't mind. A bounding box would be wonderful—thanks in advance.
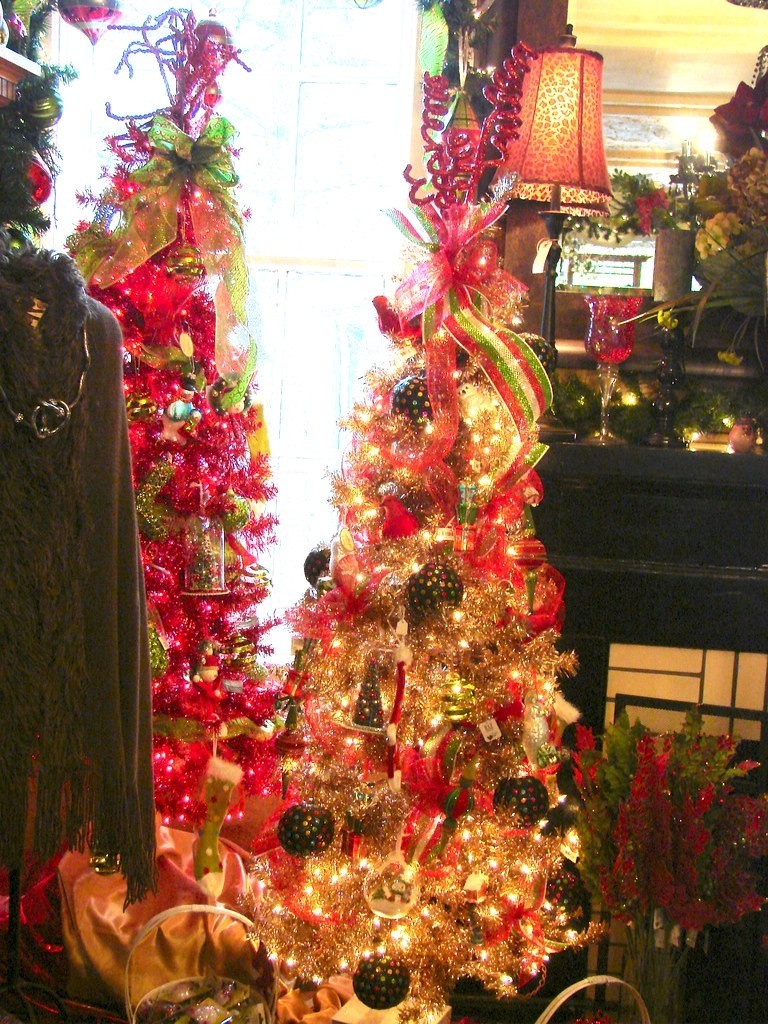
[555,292,768,390]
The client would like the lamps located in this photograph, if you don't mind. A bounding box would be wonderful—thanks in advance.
[488,24,614,441]
[669,139,718,202]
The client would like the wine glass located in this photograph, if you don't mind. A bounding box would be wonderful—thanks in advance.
[582,292,644,445]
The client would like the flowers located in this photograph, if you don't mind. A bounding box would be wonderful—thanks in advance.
[569,47,768,371]
[570,702,768,1024]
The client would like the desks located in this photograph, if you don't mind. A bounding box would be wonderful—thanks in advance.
[514,444,768,1024]
